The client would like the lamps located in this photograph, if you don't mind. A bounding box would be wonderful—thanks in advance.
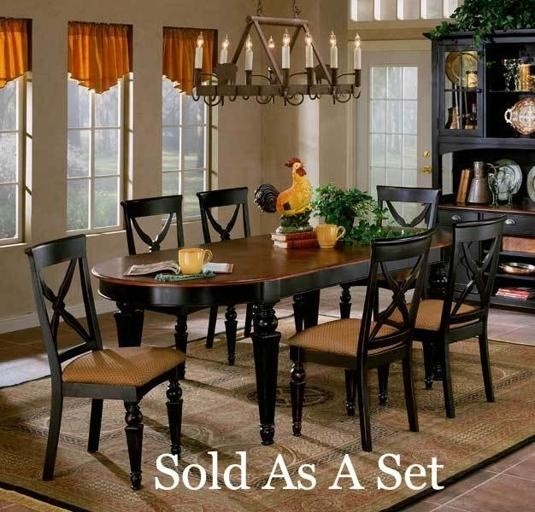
[191,0,362,106]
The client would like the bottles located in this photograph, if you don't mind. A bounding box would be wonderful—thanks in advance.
[445,103,478,128]
[466,160,492,204]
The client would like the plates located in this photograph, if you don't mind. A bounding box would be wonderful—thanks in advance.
[488,158,535,203]
[445,51,479,87]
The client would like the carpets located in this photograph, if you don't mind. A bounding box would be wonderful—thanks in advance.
[0,310,535,512]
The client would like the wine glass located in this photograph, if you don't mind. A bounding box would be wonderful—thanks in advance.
[489,172,519,209]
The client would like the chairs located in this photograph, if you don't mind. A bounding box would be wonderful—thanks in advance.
[120,187,237,364]
[195,186,303,349]
[25,234,186,491]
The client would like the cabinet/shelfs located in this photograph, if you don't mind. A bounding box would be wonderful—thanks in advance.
[421,28,535,310]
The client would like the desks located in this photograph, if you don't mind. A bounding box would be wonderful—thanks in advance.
[91,223,460,444]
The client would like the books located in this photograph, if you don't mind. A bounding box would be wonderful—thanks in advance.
[124,260,233,275]
[270,231,319,249]
[495,286,535,299]
[455,169,473,201]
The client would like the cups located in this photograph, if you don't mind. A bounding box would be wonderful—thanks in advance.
[178,248,212,275]
[517,64,532,92]
[316,223,346,248]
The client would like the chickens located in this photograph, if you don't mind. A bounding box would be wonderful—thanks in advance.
[252,157,313,218]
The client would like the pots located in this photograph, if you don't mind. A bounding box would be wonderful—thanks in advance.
[498,261,535,274]
